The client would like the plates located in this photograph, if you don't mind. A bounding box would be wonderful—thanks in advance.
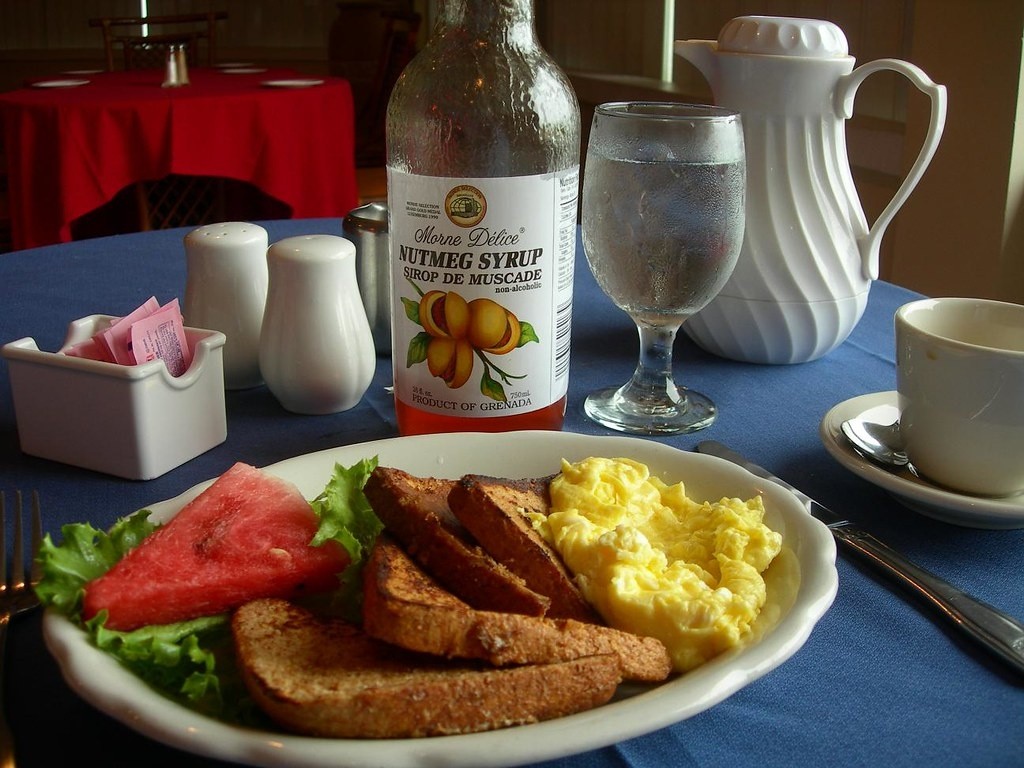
[64,70,103,73]
[32,80,89,85]
[263,80,323,85]
[820,391,1024,529]
[214,63,252,66]
[222,69,266,72]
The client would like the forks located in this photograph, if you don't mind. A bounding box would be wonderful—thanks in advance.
[0,489,44,768]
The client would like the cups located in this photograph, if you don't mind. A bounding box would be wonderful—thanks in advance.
[894,298,1024,495]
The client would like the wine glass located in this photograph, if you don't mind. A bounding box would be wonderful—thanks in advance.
[582,102,746,436]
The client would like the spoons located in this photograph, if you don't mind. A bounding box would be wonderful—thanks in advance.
[841,419,909,467]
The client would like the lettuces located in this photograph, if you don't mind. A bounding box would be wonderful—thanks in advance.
[32,456,384,718]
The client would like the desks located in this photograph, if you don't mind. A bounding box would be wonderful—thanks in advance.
[0,62,362,250]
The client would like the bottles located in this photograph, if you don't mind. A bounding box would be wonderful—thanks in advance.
[184,220,268,392]
[166,43,188,86]
[384,0,583,437]
[342,202,389,354]
[255,234,377,413]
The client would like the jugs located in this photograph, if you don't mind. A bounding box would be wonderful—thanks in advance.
[673,15,949,365]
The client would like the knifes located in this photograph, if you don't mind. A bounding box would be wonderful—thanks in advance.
[696,441,1024,670]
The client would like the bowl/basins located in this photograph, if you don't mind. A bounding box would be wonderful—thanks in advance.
[41,430,838,768]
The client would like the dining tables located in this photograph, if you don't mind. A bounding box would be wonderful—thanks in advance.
[0,219,1024,768]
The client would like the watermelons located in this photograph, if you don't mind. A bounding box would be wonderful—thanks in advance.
[83,462,350,631]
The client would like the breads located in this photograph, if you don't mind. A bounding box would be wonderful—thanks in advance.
[233,468,672,739]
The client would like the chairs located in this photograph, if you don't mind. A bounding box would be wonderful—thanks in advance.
[88,11,228,72]
